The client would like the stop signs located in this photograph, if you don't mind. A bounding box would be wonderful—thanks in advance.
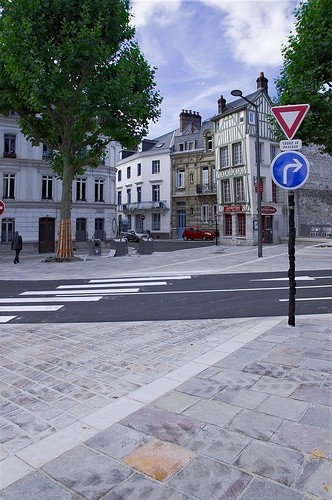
[140,215,145,220]
[0,200,6,215]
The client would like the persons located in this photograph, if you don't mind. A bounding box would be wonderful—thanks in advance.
[10,231,23,264]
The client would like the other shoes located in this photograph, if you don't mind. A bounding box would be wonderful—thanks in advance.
[17,261,19,263]
[14,261,17,264]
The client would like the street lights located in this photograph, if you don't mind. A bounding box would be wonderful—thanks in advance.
[230,89,263,257]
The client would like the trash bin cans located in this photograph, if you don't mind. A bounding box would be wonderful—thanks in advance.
[89,239,101,256]
[111,239,127,256]
[139,238,153,254]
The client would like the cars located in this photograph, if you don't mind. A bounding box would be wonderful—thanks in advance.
[182,226,215,241]
[126,229,150,243]
